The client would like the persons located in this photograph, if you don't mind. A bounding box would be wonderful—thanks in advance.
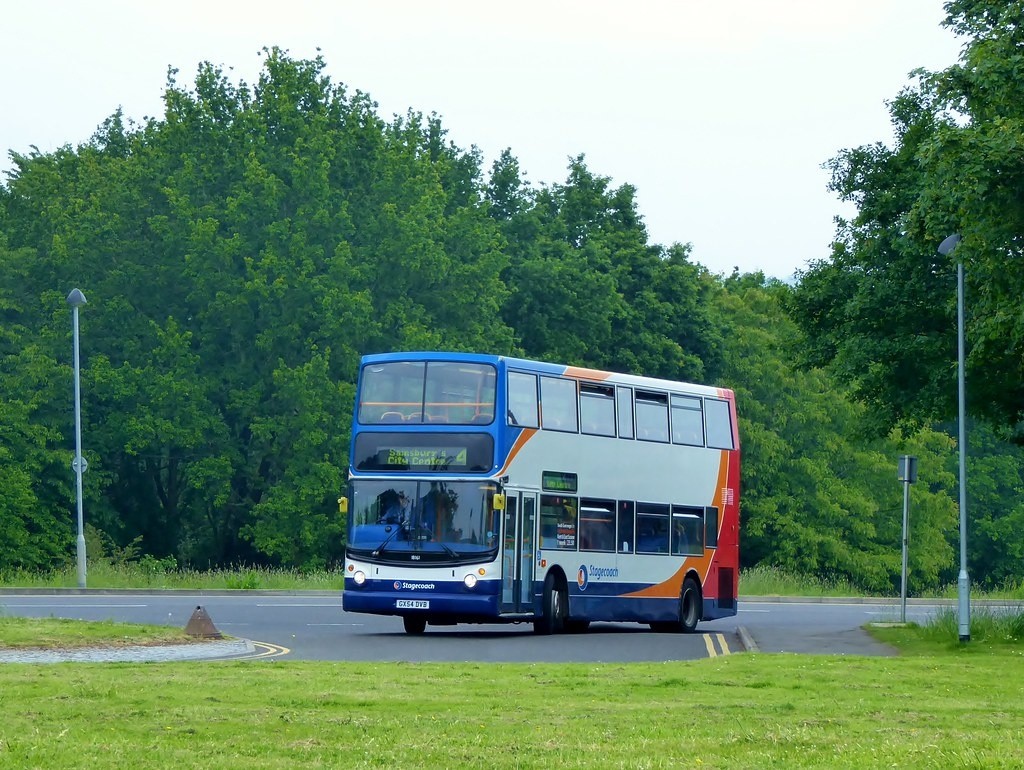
[381,493,411,523]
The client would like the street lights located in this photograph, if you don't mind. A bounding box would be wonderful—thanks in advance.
[937,233,972,641]
[66,288,88,591]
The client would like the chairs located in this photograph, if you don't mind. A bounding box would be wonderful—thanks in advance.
[380,409,716,447]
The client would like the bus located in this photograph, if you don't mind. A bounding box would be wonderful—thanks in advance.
[338,351,741,635]
